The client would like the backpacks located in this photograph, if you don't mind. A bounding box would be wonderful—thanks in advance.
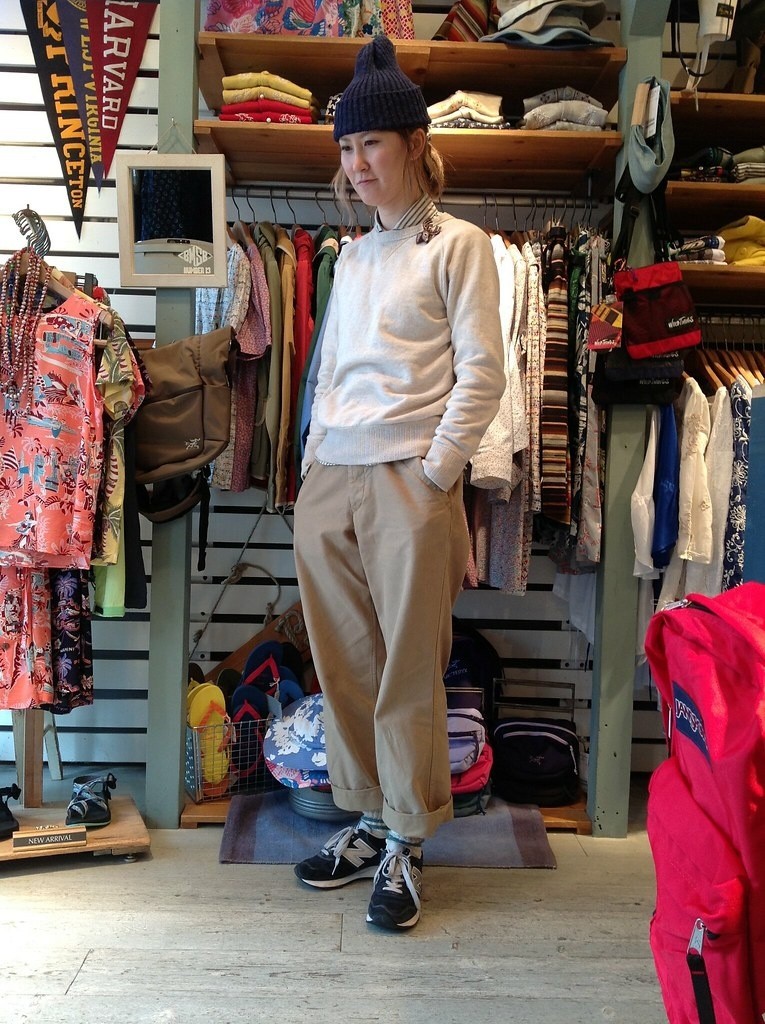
[643,580,764,1022]
[135,324,241,572]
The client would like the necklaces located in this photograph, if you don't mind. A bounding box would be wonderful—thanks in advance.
[0,246,56,420]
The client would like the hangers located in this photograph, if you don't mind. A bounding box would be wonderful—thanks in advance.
[680,313,765,395]
[0,210,114,348]
[227,183,593,247]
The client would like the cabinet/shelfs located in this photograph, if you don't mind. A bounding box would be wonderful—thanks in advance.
[195,29,627,184]
[660,90,765,294]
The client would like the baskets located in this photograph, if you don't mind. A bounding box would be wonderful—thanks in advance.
[186,717,290,804]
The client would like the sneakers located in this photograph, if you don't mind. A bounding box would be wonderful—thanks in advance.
[365,839,423,928]
[293,820,387,889]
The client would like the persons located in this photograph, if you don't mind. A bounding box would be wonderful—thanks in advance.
[294,36,506,932]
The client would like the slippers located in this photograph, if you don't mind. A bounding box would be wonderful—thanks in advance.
[186,640,306,798]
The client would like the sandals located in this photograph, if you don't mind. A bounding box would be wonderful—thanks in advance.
[0,783,22,837]
[65,772,117,828]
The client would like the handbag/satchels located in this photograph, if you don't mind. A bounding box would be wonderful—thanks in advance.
[443,616,506,730]
[612,260,701,357]
[591,346,684,407]
[492,717,583,807]
[447,707,493,819]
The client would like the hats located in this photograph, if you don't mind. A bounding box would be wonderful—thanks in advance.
[478,1,615,50]
[333,35,432,142]
[262,694,328,771]
[266,758,331,787]
